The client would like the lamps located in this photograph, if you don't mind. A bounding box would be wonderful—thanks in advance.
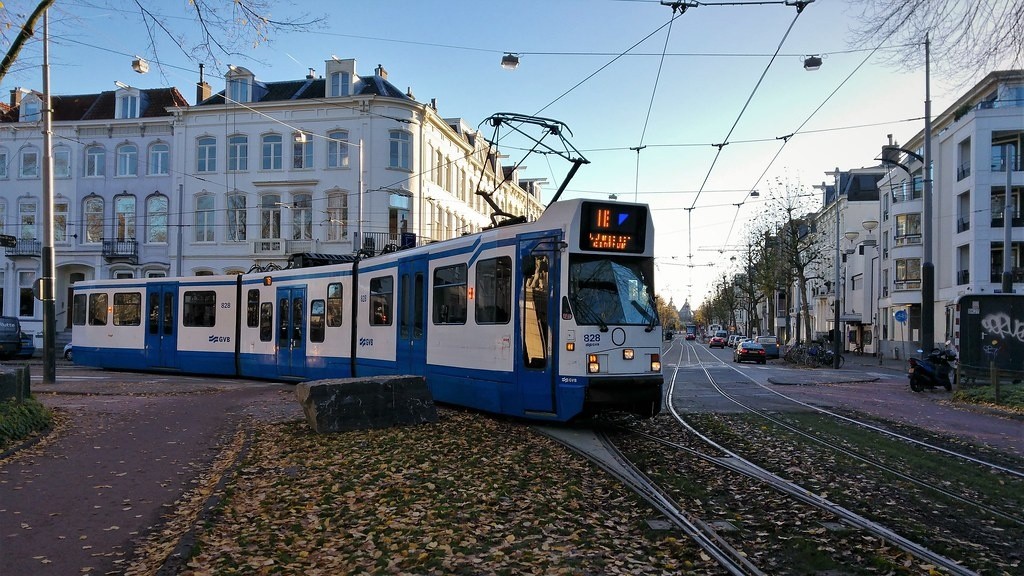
[804,56,822,71]
[862,221,879,235]
[750,191,759,199]
[845,232,859,245]
[501,54,519,70]
[294,131,306,142]
[609,194,617,201]
[131,59,149,74]
[730,257,736,261]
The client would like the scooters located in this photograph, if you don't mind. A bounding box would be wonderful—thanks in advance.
[906,340,953,393]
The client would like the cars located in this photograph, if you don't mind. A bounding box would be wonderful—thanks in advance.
[727,335,755,349]
[709,337,725,349]
[63,342,72,361]
[734,342,766,365]
[686,334,695,341]
[17,332,35,360]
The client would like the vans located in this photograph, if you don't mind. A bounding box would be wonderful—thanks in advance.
[755,336,780,359]
[715,330,728,346]
[0,316,23,360]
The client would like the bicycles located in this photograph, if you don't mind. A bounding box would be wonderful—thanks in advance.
[853,343,863,357]
[784,341,844,368]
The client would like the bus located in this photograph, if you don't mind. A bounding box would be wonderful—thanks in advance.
[71,112,664,425]
[708,324,723,338]
[686,325,696,338]
